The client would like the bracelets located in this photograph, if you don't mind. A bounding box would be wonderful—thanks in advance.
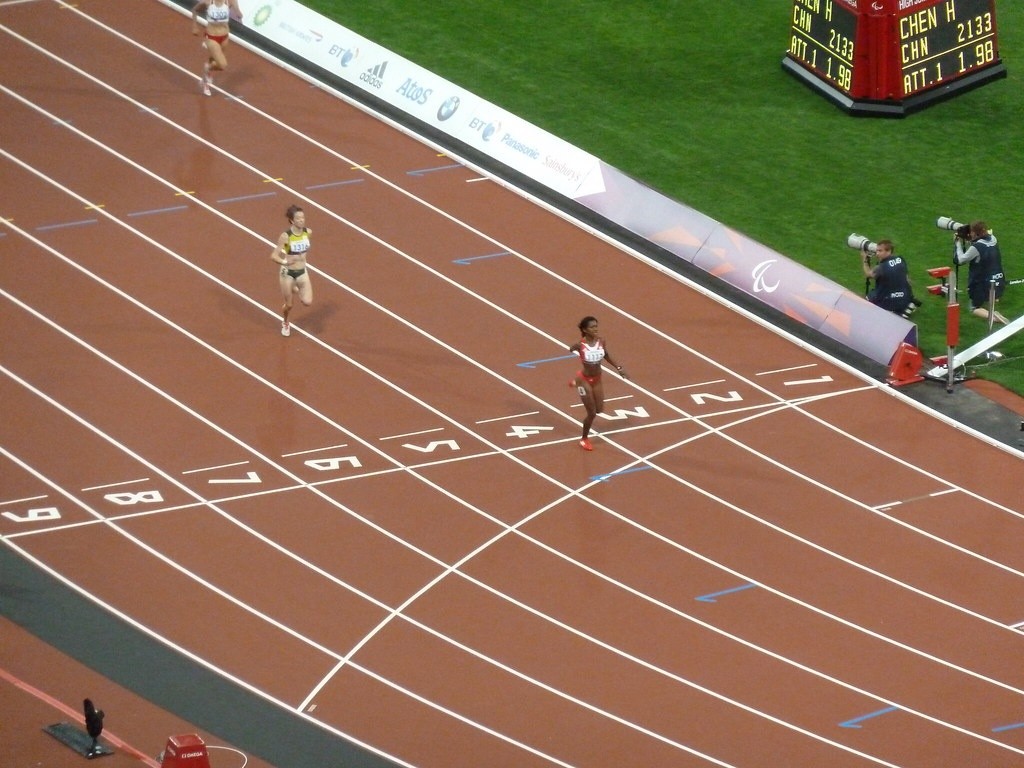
[616,365,624,372]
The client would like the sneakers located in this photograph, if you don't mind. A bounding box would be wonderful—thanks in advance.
[569,378,576,387]
[282,320,291,337]
[580,438,592,451]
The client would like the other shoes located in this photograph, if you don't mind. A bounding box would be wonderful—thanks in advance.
[204,63,213,84]
[202,83,211,96]
[994,311,1009,325]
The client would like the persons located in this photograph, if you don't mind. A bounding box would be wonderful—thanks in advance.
[953,221,1011,326]
[859,240,913,312]
[191,0,243,97]
[568,316,629,451]
[269,205,314,337]
